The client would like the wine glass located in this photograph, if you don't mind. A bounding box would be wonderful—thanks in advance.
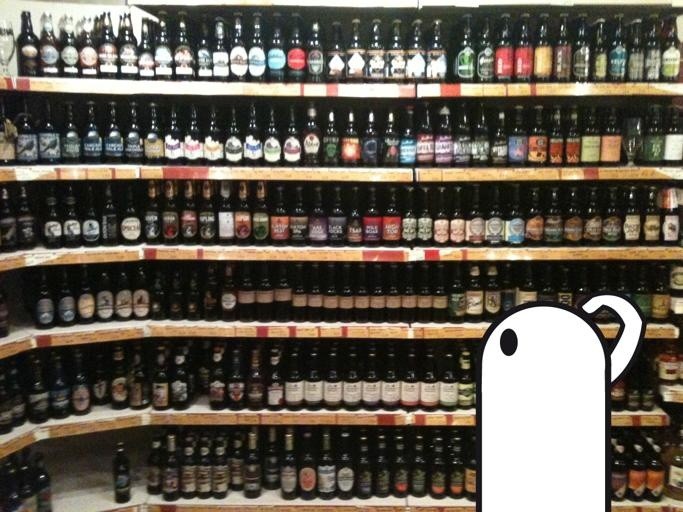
[623,117,644,167]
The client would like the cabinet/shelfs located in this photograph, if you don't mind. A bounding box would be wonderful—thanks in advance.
[0,3,681,512]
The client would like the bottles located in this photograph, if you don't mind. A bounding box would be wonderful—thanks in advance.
[109,423,683,507]
[13,4,681,84]
[0,337,683,436]
[0,444,53,512]
[0,180,683,251]
[0,89,683,168]
[0,257,682,339]
